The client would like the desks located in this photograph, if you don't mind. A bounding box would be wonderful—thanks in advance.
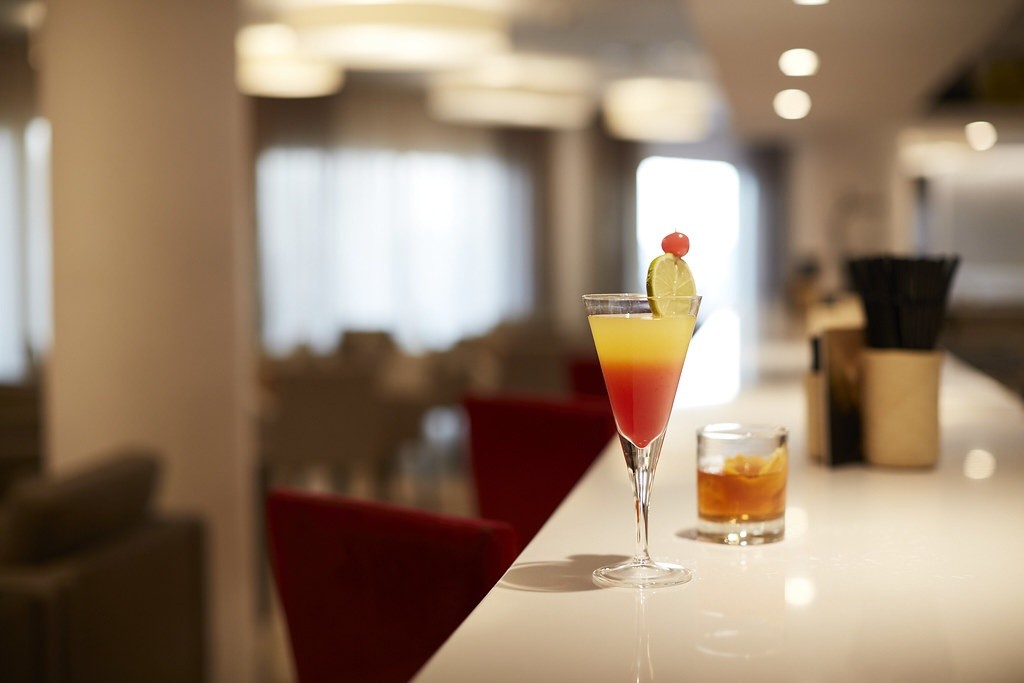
[406,310,1024,683]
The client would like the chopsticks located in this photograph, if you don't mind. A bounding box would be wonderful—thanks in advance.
[853,256,961,343]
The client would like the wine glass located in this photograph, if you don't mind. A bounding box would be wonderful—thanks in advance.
[581,291,703,589]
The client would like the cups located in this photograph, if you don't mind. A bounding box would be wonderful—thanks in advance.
[698,425,788,543]
[859,348,941,468]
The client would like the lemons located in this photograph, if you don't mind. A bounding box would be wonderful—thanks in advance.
[647,254,697,320]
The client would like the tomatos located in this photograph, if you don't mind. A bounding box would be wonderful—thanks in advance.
[662,232,689,258]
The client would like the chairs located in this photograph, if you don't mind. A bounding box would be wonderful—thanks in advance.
[0,445,202,683]
[460,391,619,549]
[258,488,519,683]
